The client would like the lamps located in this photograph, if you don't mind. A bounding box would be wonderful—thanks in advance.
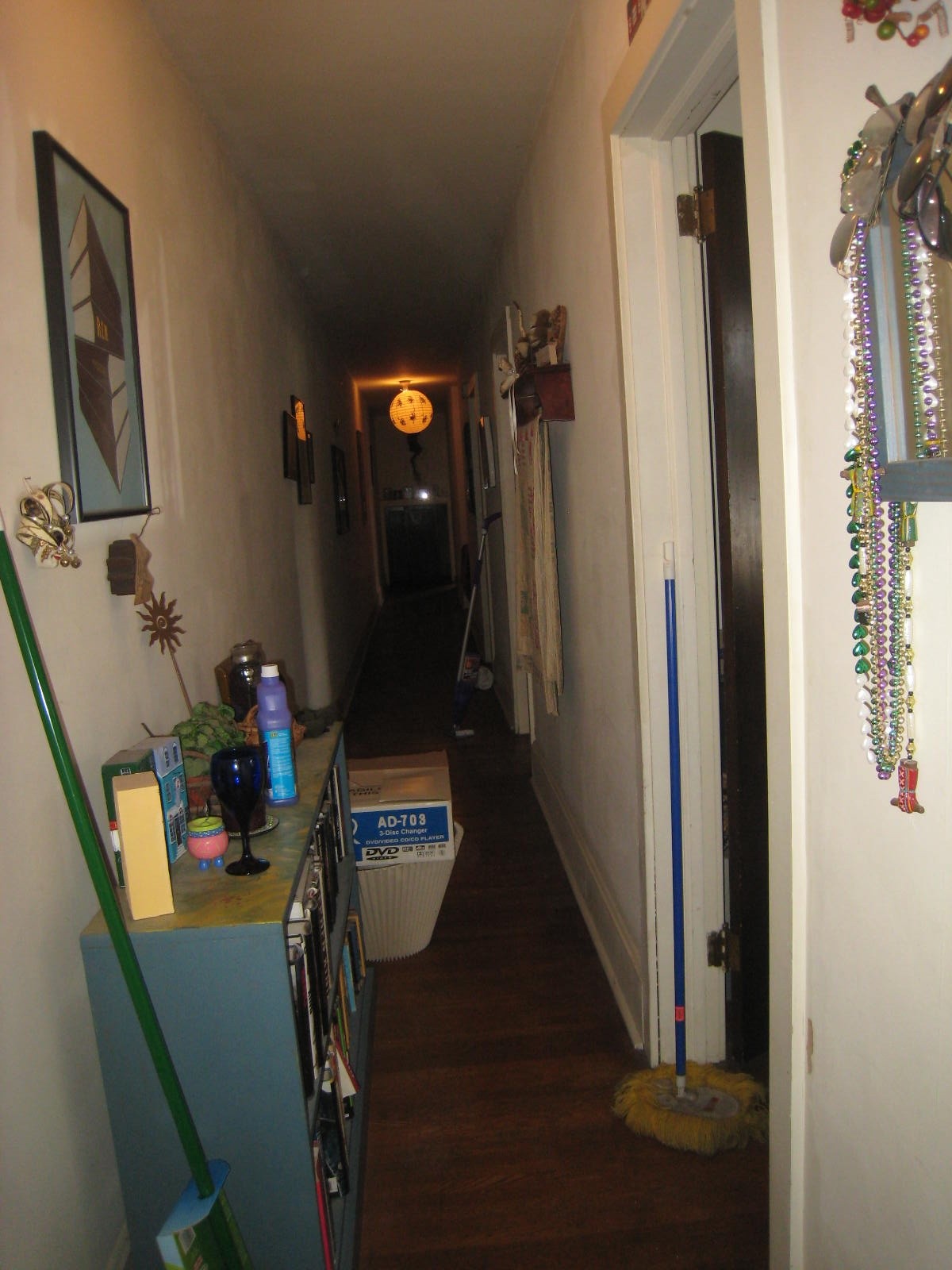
[389,380,433,435]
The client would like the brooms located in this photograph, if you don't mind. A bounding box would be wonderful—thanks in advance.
[450,522,491,727]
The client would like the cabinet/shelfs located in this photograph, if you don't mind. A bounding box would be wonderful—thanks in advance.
[81,727,377,1270]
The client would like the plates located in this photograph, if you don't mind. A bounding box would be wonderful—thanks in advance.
[228,815,280,837]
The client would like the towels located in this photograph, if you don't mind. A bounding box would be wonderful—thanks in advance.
[510,409,563,718]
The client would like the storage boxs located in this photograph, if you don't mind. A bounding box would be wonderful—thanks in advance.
[340,751,455,868]
[103,738,190,919]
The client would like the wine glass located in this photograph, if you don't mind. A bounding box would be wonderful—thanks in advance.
[210,745,272,876]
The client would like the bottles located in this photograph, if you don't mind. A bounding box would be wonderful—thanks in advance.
[229,638,264,722]
[256,664,299,808]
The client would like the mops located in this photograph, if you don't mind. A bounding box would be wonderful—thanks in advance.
[612,537,771,1157]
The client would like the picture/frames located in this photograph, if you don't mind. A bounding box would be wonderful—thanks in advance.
[33,129,157,525]
[284,411,298,482]
[330,444,350,536]
[305,432,315,484]
[290,394,313,504]
